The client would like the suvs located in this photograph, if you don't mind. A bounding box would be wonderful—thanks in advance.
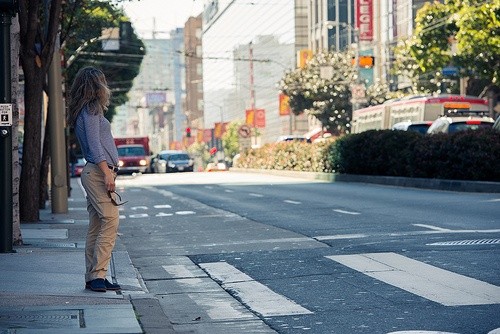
[391,118,433,135]
[425,109,495,135]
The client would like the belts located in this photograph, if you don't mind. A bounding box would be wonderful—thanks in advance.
[88,161,119,172]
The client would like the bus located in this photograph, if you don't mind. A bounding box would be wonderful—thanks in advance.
[350,94,490,135]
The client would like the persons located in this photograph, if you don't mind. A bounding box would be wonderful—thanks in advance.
[68,66,120,292]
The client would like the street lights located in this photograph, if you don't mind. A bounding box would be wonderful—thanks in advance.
[247,40,258,149]
[327,21,361,83]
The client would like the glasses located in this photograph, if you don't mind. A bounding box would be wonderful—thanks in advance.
[107,189,128,206]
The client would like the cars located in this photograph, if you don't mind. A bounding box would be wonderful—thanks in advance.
[151,151,195,172]
[69,158,86,177]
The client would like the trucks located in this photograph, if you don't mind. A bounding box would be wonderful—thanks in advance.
[113,136,154,174]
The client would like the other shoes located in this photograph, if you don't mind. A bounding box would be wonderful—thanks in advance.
[86,278,120,289]
[89,278,106,292]
